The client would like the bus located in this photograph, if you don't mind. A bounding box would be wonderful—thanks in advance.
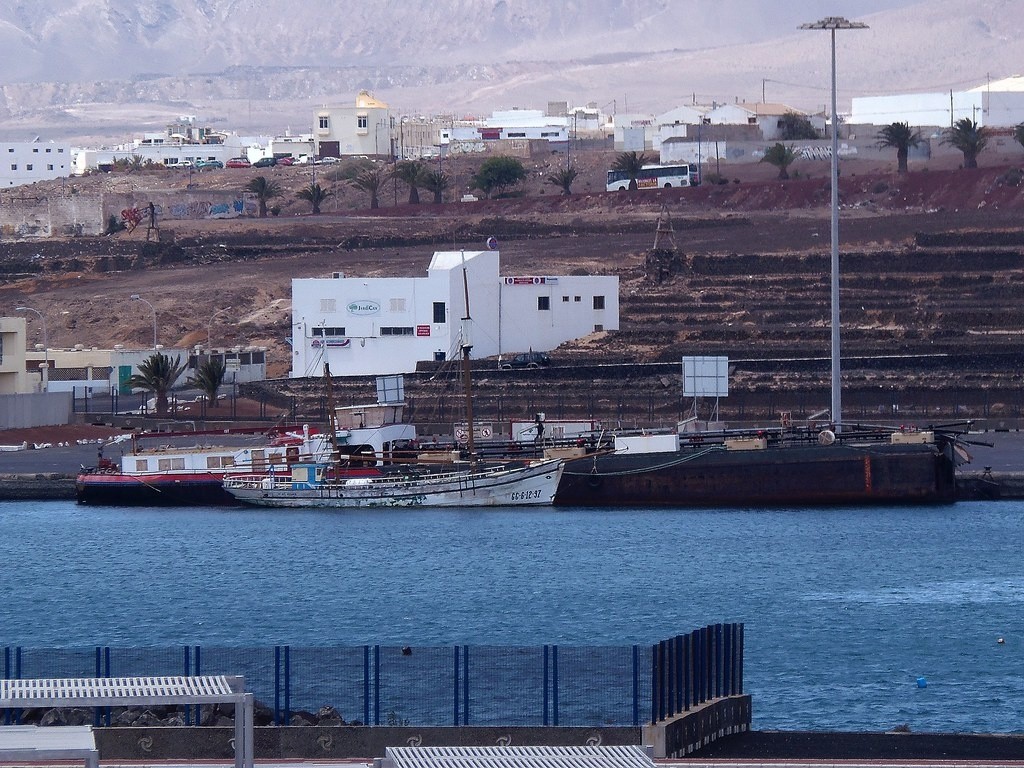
[603,162,700,192]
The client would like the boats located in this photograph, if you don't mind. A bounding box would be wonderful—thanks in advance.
[72,423,386,505]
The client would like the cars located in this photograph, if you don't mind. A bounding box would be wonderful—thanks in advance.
[165,155,369,170]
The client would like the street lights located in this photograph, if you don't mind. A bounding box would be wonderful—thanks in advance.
[14,306,49,393]
[208,303,233,365]
[131,294,157,355]
[796,15,872,443]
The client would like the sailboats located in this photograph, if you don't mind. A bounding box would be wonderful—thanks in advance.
[219,325,631,508]
